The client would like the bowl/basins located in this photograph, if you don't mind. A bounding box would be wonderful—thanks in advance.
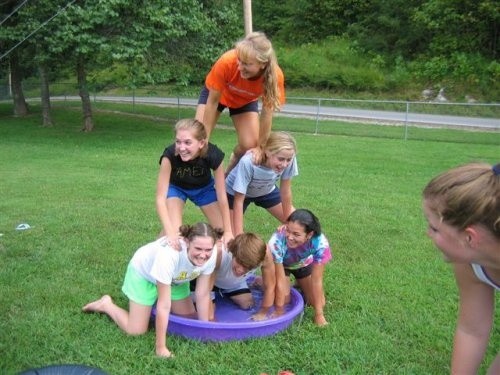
[153,275,304,342]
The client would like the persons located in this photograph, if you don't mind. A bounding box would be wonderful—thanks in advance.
[225,132,298,238]
[422,163,500,375]
[252,209,332,327]
[82,222,224,358]
[156,118,234,252]
[194,32,285,179]
[190,233,276,321]
